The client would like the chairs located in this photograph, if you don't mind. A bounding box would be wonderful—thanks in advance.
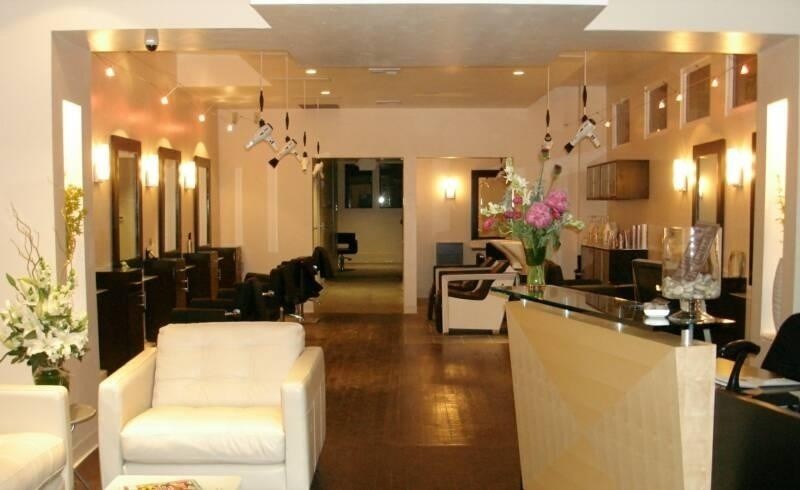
[760,313,800,381]
[427,242,523,336]
[167,247,328,323]
[336,232,358,272]
[1,385,74,490]
[98,321,327,490]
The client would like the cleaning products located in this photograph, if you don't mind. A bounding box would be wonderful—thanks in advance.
[631,223,648,248]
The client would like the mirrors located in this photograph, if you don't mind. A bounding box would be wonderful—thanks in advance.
[158,146,182,258]
[193,155,212,253]
[111,134,143,268]
[471,169,511,240]
[692,139,726,228]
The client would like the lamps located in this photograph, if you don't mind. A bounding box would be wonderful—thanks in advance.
[93,144,110,182]
[443,176,457,198]
[672,159,688,192]
[146,155,158,188]
[184,160,198,190]
[727,148,743,186]
[698,176,703,199]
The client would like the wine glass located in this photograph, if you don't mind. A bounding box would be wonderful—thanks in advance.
[661,224,723,326]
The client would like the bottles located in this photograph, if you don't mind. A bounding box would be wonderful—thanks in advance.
[589,214,629,250]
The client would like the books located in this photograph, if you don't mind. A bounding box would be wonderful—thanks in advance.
[713,376,759,389]
[122,478,202,490]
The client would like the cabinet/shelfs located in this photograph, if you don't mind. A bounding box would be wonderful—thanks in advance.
[580,244,648,285]
[585,159,650,200]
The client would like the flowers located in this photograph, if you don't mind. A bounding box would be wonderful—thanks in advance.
[2,199,90,368]
[481,155,585,295]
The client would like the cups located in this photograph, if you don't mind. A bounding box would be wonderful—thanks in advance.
[629,224,649,249]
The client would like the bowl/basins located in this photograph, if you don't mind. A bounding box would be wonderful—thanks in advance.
[642,309,671,316]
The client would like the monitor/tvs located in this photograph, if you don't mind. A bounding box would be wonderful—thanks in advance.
[631,258,663,303]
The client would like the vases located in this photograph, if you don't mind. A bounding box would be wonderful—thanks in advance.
[520,246,551,297]
[33,365,69,390]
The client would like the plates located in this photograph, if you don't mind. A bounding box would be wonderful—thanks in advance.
[644,317,669,327]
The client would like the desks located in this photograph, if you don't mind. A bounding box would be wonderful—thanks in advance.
[69,400,95,490]
[104,473,242,490]
